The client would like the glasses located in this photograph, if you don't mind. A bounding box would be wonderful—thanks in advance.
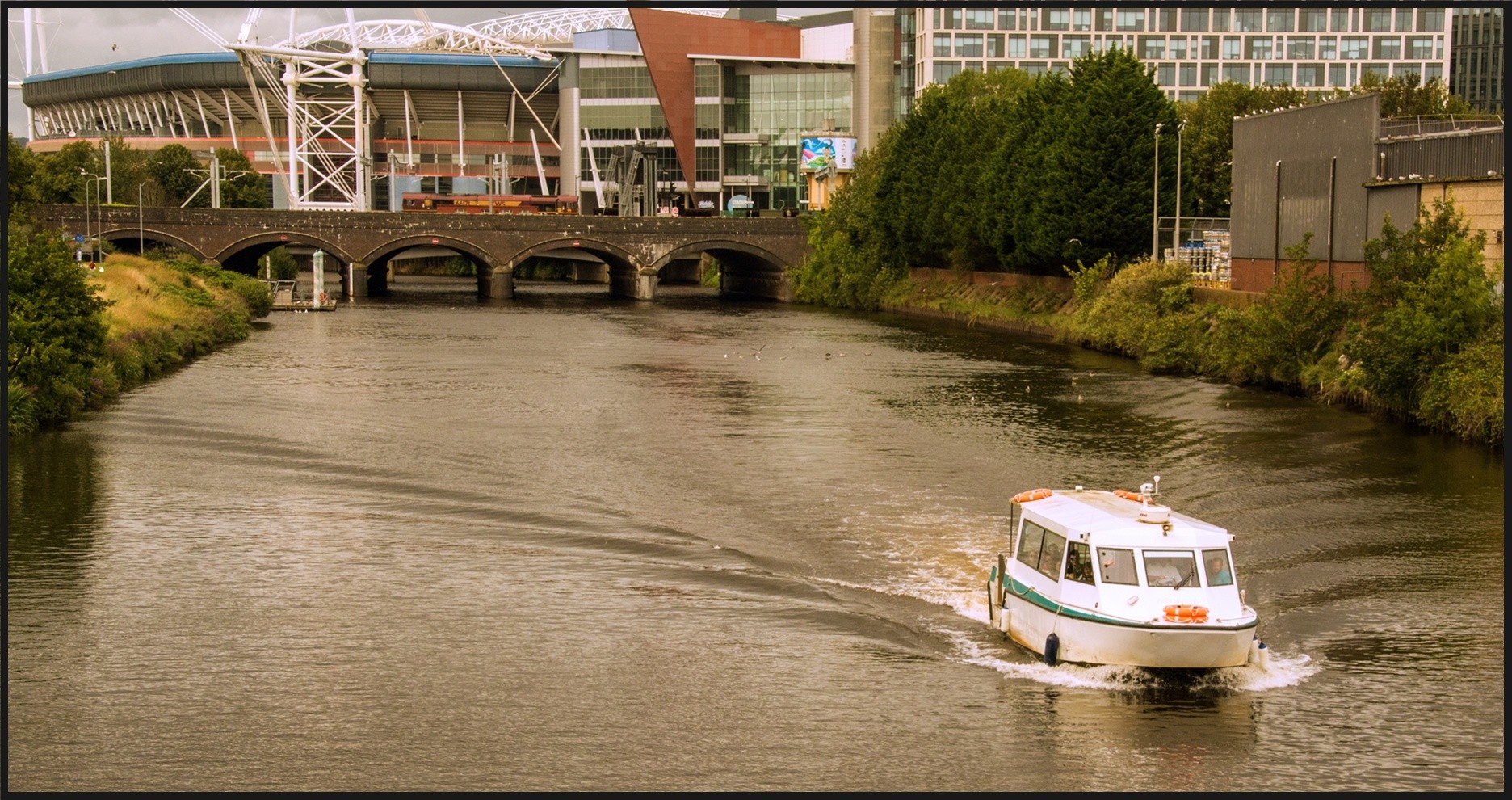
[1029,555,1036,558]
[1071,557,1077,560]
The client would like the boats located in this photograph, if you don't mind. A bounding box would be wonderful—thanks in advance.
[987,476,1260,684]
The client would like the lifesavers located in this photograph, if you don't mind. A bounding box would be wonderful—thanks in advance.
[1114,489,1153,503]
[1164,604,1210,623]
[1013,489,1052,502]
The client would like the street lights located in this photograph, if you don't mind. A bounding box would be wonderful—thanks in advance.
[82,172,102,263]
[86,177,108,238]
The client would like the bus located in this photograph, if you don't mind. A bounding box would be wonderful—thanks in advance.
[402,193,580,216]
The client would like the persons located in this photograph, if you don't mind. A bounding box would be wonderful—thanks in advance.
[1206,558,1230,584]
[89,261,96,272]
[1025,542,1062,579]
[1067,550,1094,581]
[1146,558,1183,586]
